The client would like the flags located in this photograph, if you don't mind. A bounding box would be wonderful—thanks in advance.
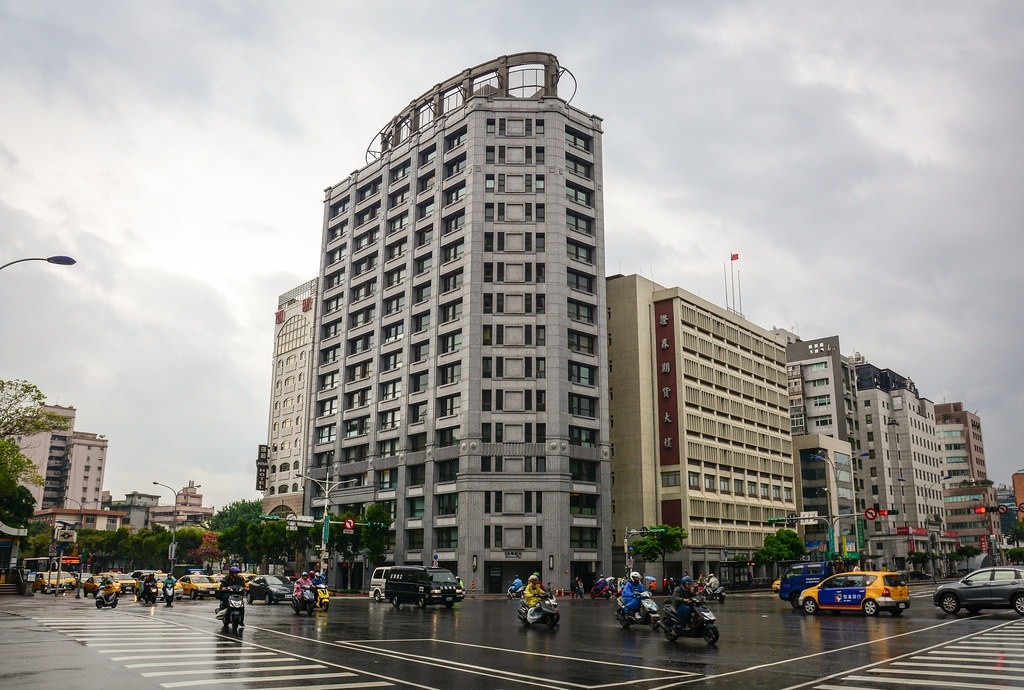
[731,254,739,261]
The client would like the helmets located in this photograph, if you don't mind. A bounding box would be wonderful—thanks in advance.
[229,566,240,574]
[315,572,321,576]
[630,572,642,581]
[302,571,307,576]
[682,576,693,585]
[168,572,172,576]
[533,572,539,575]
[149,572,154,575]
[528,575,538,581]
[309,570,315,574]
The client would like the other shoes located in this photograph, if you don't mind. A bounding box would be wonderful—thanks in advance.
[682,626,691,630]
[635,612,642,618]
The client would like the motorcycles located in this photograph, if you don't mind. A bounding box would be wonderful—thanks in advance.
[507,581,526,600]
[517,593,560,628]
[94,585,119,609]
[657,595,720,644]
[591,579,612,599]
[144,579,159,604]
[615,591,661,630]
[691,579,726,602]
[368,565,467,610]
[221,575,252,630]
[290,581,331,615]
[162,581,177,606]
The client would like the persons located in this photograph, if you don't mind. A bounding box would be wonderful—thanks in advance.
[99,572,176,601]
[293,570,326,602]
[220,567,248,626]
[510,572,548,610]
[573,572,720,630]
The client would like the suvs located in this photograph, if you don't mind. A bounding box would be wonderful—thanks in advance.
[799,570,911,616]
[932,566,1024,616]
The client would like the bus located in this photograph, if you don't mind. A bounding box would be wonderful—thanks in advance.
[23,556,83,584]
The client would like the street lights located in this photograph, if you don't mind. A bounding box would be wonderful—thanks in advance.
[152,481,202,576]
[628,546,634,573]
[896,476,953,580]
[296,473,359,583]
[814,450,873,556]
[724,548,729,579]
[63,495,110,541]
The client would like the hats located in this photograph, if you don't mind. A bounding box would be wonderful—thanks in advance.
[708,574,714,576]
[600,574,603,576]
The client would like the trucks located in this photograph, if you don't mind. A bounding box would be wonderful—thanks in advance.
[779,560,835,608]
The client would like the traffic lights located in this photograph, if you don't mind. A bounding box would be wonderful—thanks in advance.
[973,506,996,514]
[368,521,387,528]
[877,509,899,516]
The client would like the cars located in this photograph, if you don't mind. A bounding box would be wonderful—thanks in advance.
[81,569,298,604]
[772,578,781,592]
